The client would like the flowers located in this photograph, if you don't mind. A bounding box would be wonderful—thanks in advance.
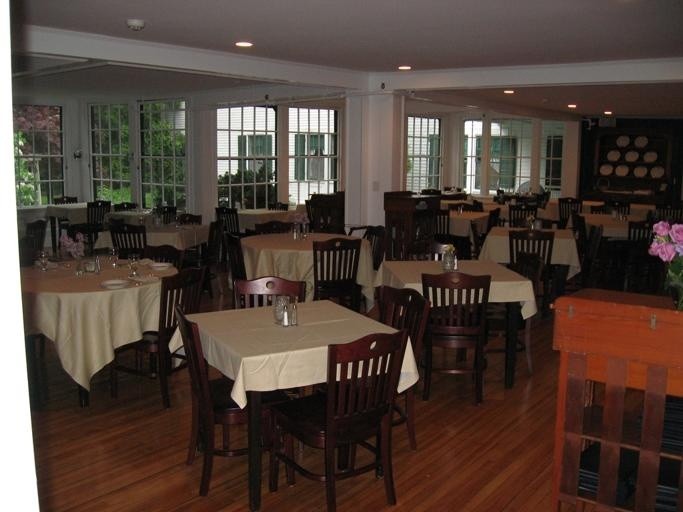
[648,220,683,283]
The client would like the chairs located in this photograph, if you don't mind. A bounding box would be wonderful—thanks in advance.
[488,207,500,228]
[420,272,492,406]
[590,196,630,215]
[19,234,38,268]
[233,278,306,305]
[191,220,224,298]
[401,239,452,261]
[225,232,246,280]
[174,303,278,497]
[615,221,653,291]
[80,200,111,255]
[268,202,288,212]
[313,238,362,315]
[657,202,683,224]
[363,225,388,271]
[53,196,78,249]
[153,206,177,224]
[174,214,203,226]
[448,200,483,213]
[575,223,603,277]
[109,264,206,409]
[470,220,481,256]
[225,207,241,235]
[270,328,408,512]
[493,189,551,208]
[305,191,344,233]
[559,197,583,230]
[214,207,225,222]
[26,220,47,256]
[436,210,449,241]
[509,203,537,230]
[487,252,545,376]
[114,202,137,212]
[508,229,554,318]
[144,245,183,267]
[109,222,146,261]
[318,285,429,468]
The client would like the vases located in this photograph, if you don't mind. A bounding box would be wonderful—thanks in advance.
[670,284,683,309]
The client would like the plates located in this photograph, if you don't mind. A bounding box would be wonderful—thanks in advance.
[101,280,127,289]
[119,259,128,265]
[153,262,173,270]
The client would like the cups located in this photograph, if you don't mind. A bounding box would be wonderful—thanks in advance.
[303,224,308,234]
[291,227,296,242]
[64,263,72,272]
[113,259,120,272]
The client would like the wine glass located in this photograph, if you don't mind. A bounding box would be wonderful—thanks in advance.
[40,250,48,271]
[128,253,140,276]
